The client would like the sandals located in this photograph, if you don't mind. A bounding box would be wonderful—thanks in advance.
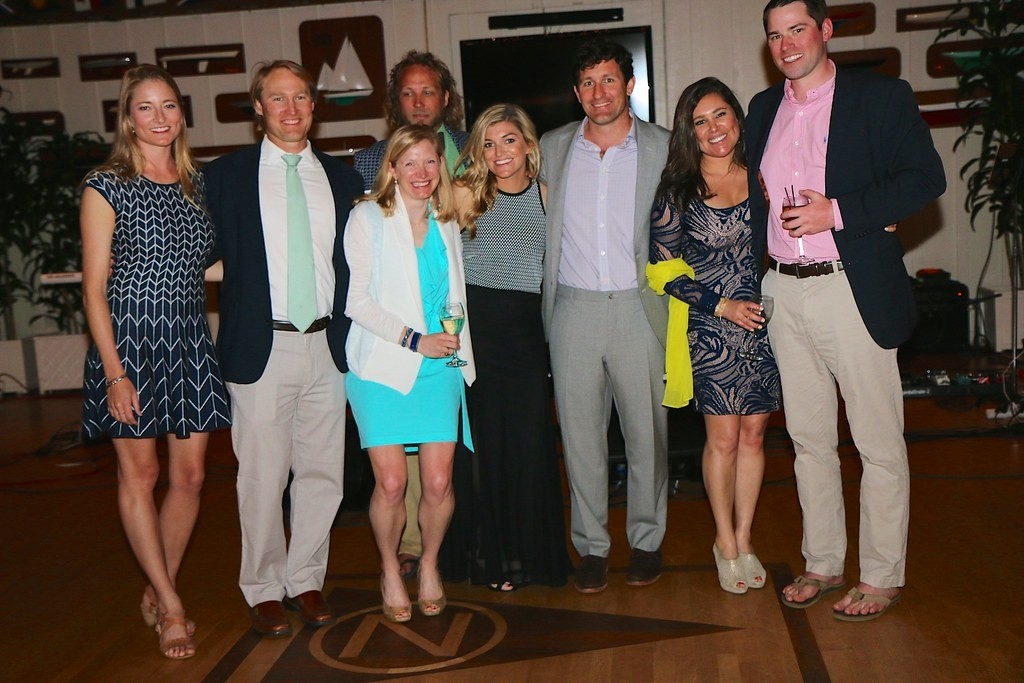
[487,581,517,592]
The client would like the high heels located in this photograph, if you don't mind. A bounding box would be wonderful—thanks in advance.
[712,544,748,593]
[380,573,412,622]
[417,563,446,617]
[140,583,197,636]
[737,549,766,589]
[155,605,196,660]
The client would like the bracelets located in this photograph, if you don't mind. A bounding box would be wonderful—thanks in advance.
[402,328,422,352]
[715,298,729,317]
[107,374,128,386]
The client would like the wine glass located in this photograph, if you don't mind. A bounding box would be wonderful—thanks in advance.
[783,194,815,266]
[739,294,774,360]
[438,302,468,368]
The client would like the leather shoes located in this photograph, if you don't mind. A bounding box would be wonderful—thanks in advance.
[251,600,292,637]
[282,590,334,625]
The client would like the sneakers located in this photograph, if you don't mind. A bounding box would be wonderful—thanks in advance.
[625,548,662,586]
[573,555,609,593]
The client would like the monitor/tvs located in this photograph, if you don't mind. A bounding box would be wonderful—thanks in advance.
[460,25,656,140]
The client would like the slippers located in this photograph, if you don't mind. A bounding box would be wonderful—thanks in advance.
[831,587,903,622]
[781,575,847,609]
[400,558,419,579]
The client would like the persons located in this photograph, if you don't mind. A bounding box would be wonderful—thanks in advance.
[353,51,472,581]
[353,103,572,592]
[109,59,366,637]
[538,42,669,593]
[645,77,782,594]
[342,121,477,620]
[79,62,232,658]
[741,0,946,622]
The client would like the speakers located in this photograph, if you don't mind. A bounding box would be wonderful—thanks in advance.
[902,279,969,354]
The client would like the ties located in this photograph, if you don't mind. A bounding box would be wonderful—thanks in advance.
[281,153,318,332]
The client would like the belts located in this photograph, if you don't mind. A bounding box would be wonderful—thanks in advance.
[273,315,330,333]
[768,256,844,279]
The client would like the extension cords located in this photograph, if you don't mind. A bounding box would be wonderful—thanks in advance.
[986,403,1019,420]
